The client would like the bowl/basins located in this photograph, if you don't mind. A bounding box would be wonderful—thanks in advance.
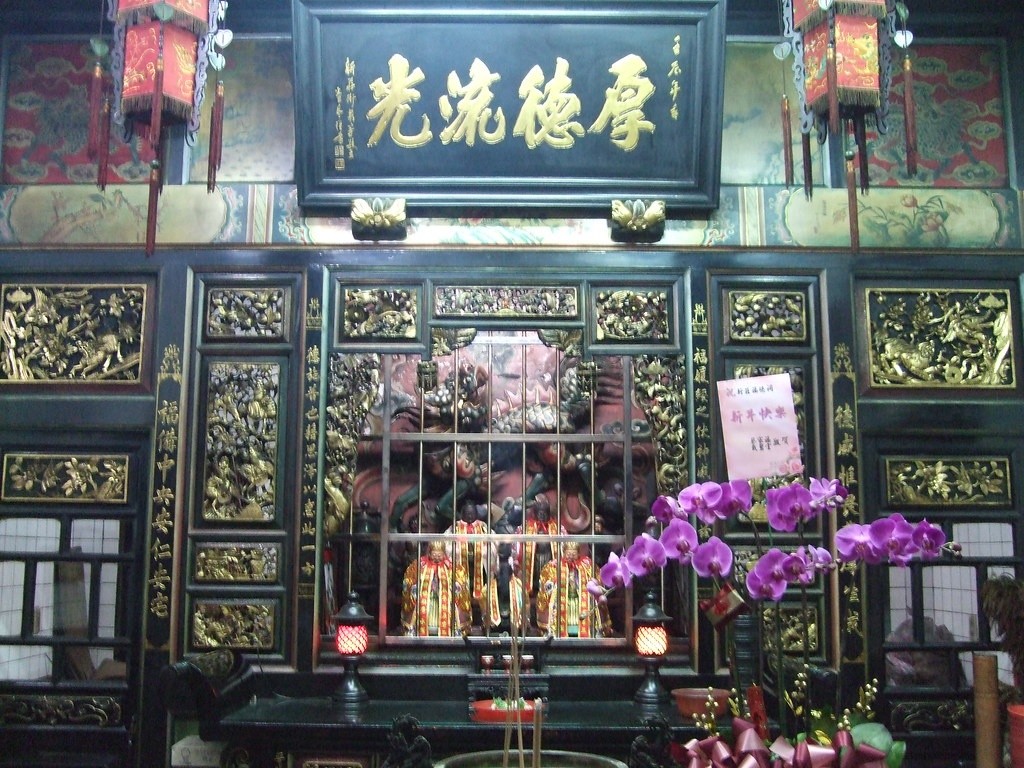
[473,699,545,723]
[671,687,733,719]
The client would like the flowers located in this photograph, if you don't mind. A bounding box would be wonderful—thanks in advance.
[579,478,948,768]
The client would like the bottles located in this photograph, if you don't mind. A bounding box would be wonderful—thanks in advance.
[481,656,495,674]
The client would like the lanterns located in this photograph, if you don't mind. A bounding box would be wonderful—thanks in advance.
[773,0,918,248]
[87,0,234,259]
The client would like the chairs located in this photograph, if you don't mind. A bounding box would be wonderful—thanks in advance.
[56,544,125,684]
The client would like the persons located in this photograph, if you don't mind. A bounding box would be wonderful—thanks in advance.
[511,501,568,596]
[444,500,500,578]
[536,542,613,637]
[401,540,470,636]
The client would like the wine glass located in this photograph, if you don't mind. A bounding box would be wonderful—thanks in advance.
[521,654,535,674]
[501,655,514,674]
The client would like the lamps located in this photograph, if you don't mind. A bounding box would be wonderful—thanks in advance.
[327,589,376,702]
[628,587,673,704]
[773,0,919,255]
[89,0,236,256]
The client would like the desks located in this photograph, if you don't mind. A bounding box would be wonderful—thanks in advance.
[156,649,839,768]
